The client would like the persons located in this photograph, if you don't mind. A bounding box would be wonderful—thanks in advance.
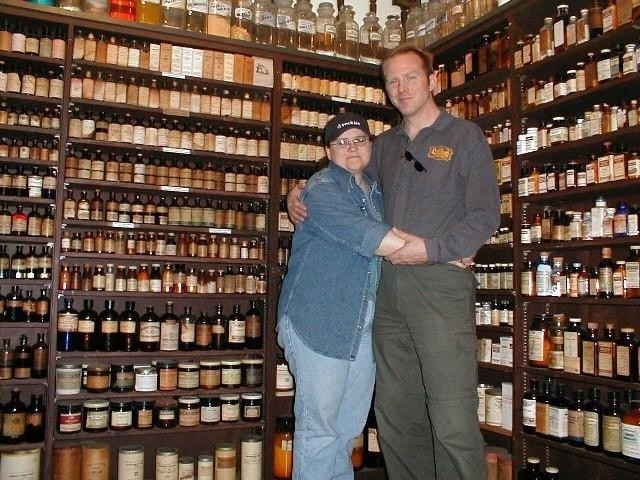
[284,45,503,480]
[273,109,479,480]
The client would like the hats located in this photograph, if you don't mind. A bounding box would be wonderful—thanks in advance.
[322,112,372,144]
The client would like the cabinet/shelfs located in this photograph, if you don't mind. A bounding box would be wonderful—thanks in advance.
[0,1,401,480]
[424,1,640,479]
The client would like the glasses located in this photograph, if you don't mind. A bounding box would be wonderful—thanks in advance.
[404,150,427,174]
[327,135,372,148]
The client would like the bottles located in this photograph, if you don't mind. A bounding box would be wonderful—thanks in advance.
[58,70,270,354]
[517,0,640,480]
[432,1,509,145]
[276,418,382,476]
[471,226,514,327]
[1,20,64,377]
[281,64,395,161]
[279,165,314,285]
[3,387,26,444]
[26,393,45,442]
[57,0,430,64]
